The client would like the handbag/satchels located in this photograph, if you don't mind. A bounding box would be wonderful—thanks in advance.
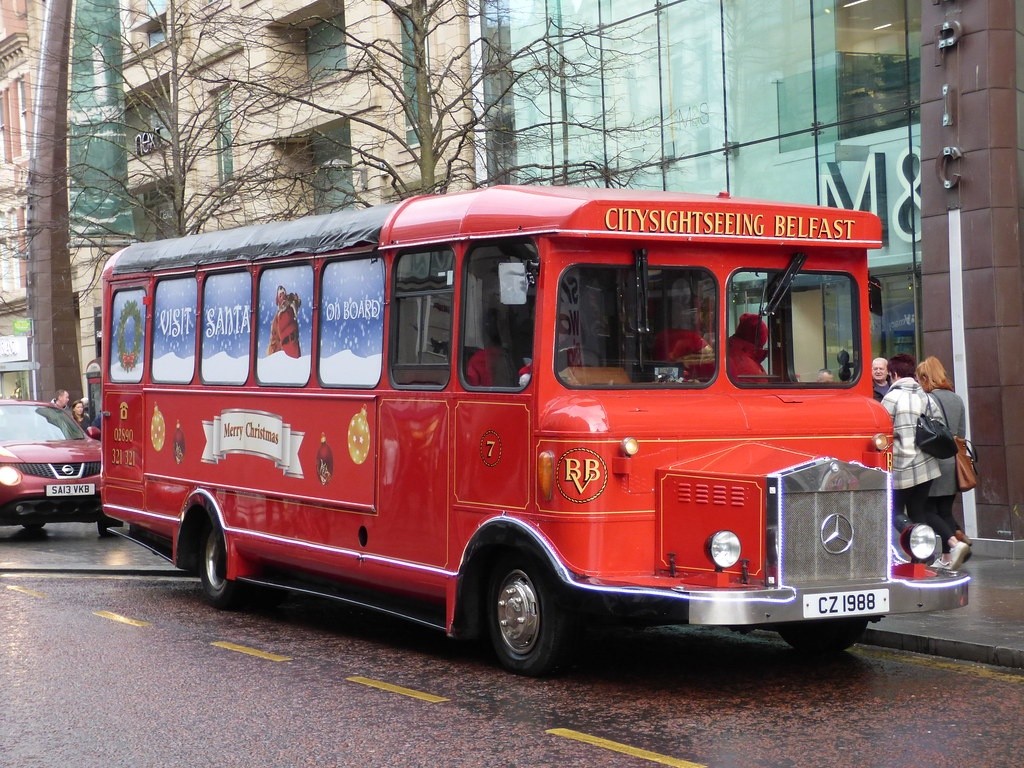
[916,392,958,459]
[952,435,978,492]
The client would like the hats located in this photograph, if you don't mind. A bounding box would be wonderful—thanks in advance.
[735,313,768,348]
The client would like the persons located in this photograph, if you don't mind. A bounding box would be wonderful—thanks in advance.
[728,311,768,384]
[815,368,834,384]
[653,297,715,383]
[464,308,520,387]
[10,379,22,399]
[49,389,101,431]
[870,352,972,573]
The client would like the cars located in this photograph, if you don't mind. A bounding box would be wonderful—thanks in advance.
[0,398,123,537]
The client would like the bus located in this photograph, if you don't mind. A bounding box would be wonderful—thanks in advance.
[98,184,892,680]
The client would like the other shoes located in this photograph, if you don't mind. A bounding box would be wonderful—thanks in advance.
[929,556,952,569]
[950,541,969,571]
[955,530,973,564]
